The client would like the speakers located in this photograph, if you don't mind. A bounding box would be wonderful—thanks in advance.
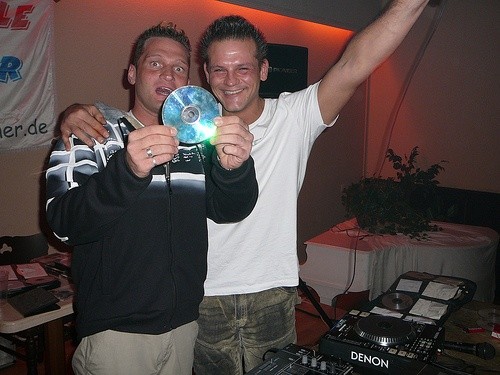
[256,42,308,99]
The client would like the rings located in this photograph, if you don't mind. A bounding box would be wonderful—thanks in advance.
[152,156,157,166]
[146,148,152,157]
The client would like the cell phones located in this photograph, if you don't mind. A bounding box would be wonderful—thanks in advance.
[47,262,72,281]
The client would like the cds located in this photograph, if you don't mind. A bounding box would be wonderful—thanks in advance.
[162,85,220,144]
[383,293,413,310]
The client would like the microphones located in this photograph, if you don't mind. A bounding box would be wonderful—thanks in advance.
[0,280,60,298]
[442,341,496,361]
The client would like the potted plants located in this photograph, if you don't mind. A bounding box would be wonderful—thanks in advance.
[341,146,448,241]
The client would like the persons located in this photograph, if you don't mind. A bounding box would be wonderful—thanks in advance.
[60,0,428,375]
[45,26,258,375]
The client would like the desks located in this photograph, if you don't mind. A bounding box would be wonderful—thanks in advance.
[0,256,75,375]
[443,300,500,375]
